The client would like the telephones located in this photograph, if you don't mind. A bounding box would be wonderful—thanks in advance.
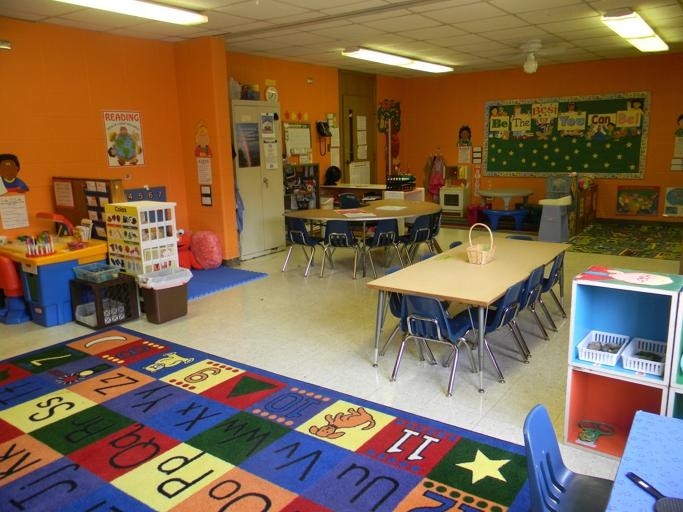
[317,120,332,137]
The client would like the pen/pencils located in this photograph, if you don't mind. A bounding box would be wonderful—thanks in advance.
[24,233,55,256]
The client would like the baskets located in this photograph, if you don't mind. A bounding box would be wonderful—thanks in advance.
[465,222,497,265]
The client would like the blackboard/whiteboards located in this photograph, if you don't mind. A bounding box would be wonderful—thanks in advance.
[481,90,650,179]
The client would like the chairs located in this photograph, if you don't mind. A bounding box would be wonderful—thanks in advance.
[399,214,432,260]
[441,281,529,384]
[411,208,443,256]
[380,265,437,364]
[507,236,535,242]
[448,239,463,249]
[391,293,478,396]
[281,219,335,276]
[319,220,368,280]
[521,402,616,511]
[418,253,437,259]
[356,218,404,275]
[542,250,567,319]
[519,264,558,342]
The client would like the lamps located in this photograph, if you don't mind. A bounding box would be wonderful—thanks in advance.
[55,0,209,28]
[600,8,672,54]
[522,37,543,74]
[342,47,457,76]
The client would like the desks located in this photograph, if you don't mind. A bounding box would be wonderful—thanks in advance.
[280,199,442,277]
[479,187,534,211]
[0,232,108,327]
[605,408,682,511]
[364,235,573,394]
[321,183,388,205]
[479,210,529,230]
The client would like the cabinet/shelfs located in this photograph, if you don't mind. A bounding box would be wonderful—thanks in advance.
[439,165,472,217]
[562,367,668,461]
[569,264,682,386]
[670,393,683,421]
[285,163,322,240]
[669,292,683,389]
[230,99,289,259]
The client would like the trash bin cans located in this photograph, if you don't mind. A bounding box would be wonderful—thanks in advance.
[137,265,194,325]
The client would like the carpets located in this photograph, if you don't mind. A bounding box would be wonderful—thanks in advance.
[563,221,683,261]
[0,326,533,512]
[186,255,269,302]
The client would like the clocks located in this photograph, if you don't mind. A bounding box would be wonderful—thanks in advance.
[264,85,280,103]
